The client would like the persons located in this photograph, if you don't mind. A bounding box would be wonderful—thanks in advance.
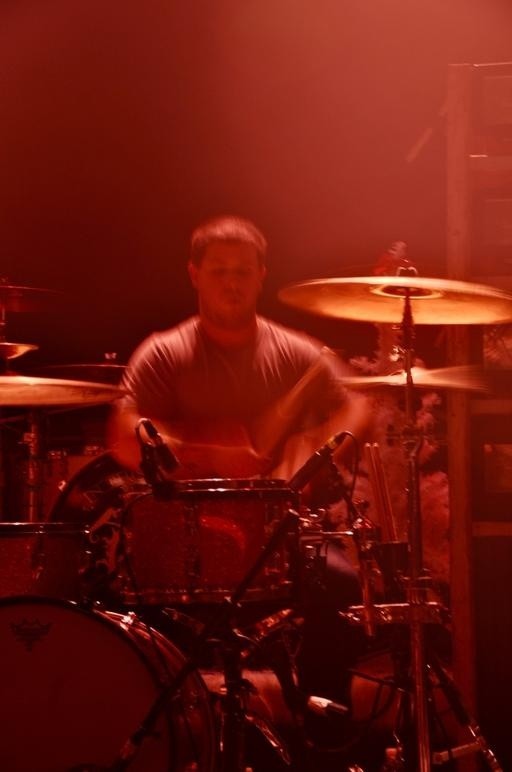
[108,215,368,771]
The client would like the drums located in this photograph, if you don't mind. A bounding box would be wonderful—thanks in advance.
[0,594,220,772]
[0,521,104,610]
[105,477,302,608]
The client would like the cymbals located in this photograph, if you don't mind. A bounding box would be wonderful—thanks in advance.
[0,376,128,405]
[0,340,40,361]
[278,276,512,326]
[334,365,480,391]
[0,282,66,314]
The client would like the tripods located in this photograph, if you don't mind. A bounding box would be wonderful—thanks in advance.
[386,298,498,771]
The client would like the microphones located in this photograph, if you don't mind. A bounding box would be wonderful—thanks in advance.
[285,430,351,492]
[142,418,179,475]
